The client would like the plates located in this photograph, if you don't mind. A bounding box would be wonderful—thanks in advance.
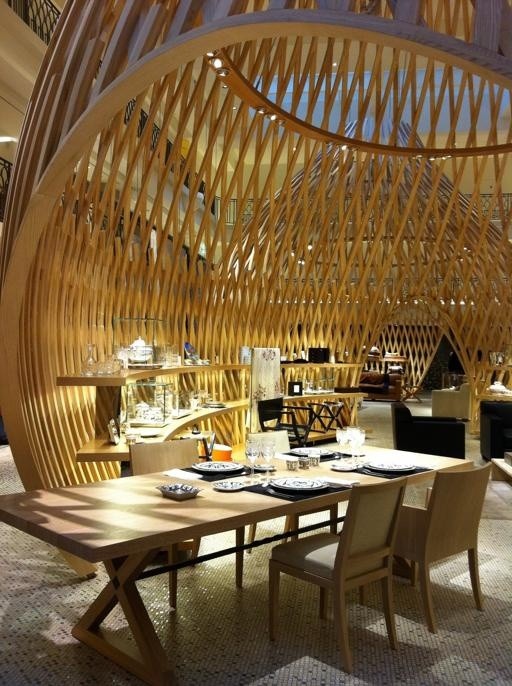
[330,461,364,471]
[362,459,417,471]
[125,427,161,437]
[208,403,225,408]
[243,464,278,472]
[191,461,244,471]
[212,481,245,492]
[269,477,330,491]
[289,448,335,457]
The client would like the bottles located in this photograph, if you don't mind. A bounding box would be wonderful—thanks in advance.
[82,344,97,376]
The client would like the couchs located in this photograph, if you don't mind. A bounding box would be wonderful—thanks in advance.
[334,372,403,401]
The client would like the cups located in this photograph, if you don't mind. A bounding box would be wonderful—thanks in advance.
[198,390,207,407]
[96,358,122,376]
[308,449,321,467]
[298,458,309,470]
[286,460,298,471]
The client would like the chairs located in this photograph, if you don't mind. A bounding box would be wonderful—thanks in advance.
[246,430,338,554]
[358,462,492,634]
[258,397,313,447]
[269,475,407,674]
[432,383,477,421]
[480,400,512,462]
[391,402,465,459]
[129,439,201,566]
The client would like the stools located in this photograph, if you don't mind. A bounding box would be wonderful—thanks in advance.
[401,385,422,402]
[307,401,344,433]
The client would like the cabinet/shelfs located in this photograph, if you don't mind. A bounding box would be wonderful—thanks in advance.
[55,362,370,477]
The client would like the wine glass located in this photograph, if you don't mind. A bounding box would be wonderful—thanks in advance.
[259,438,277,487]
[245,438,261,486]
[336,425,366,464]
